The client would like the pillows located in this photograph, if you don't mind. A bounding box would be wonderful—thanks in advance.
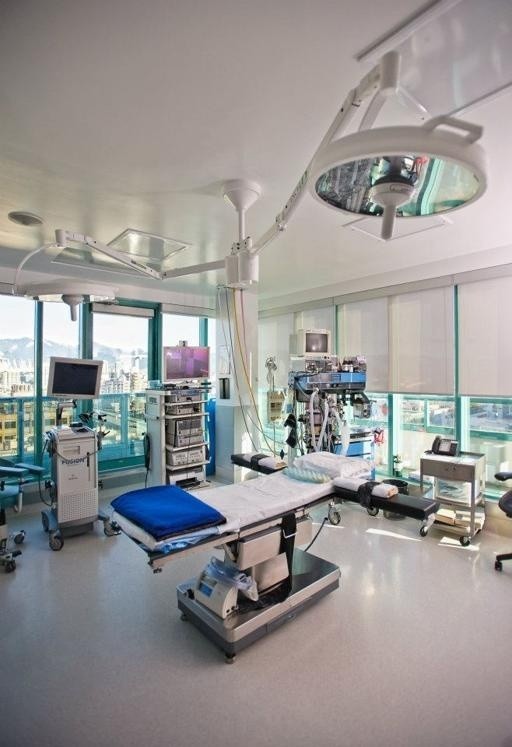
[293,450,373,479]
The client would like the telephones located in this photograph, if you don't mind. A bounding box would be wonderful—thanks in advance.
[432,435,458,456]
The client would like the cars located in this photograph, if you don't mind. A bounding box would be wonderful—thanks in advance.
[434,403,512,432]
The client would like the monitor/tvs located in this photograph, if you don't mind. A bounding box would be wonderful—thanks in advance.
[164,345,210,382]
[297,329,331,355]
[47,357,103,400]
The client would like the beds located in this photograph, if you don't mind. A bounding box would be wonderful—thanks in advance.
[112,450,374,664]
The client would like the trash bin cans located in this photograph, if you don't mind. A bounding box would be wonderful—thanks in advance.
[382,479,408,520]
